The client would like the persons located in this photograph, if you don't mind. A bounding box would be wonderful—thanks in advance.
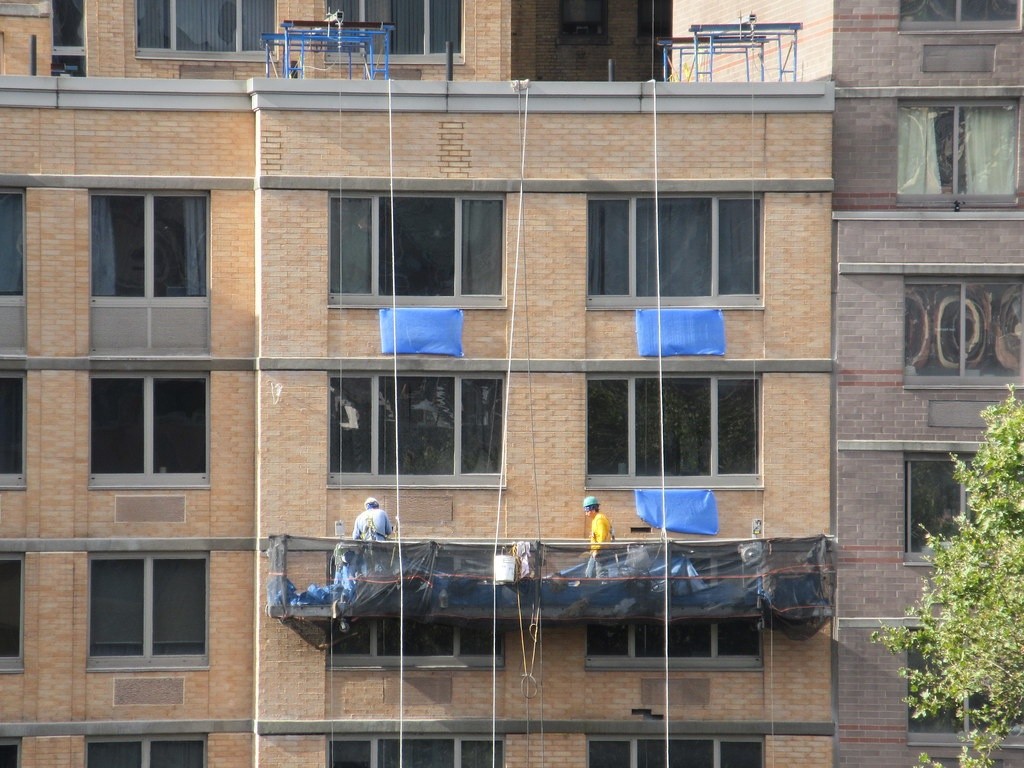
[353,497,391,572]
[583,496,615,578]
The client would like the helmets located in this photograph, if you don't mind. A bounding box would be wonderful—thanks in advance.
[583,496,601,507]
[364,497,380,511]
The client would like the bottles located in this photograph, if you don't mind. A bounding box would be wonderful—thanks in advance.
[439,588,448,607]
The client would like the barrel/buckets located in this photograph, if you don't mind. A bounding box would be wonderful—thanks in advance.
[494,546,516,582]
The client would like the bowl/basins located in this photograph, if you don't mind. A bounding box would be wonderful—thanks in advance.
[502,585,524,602]
